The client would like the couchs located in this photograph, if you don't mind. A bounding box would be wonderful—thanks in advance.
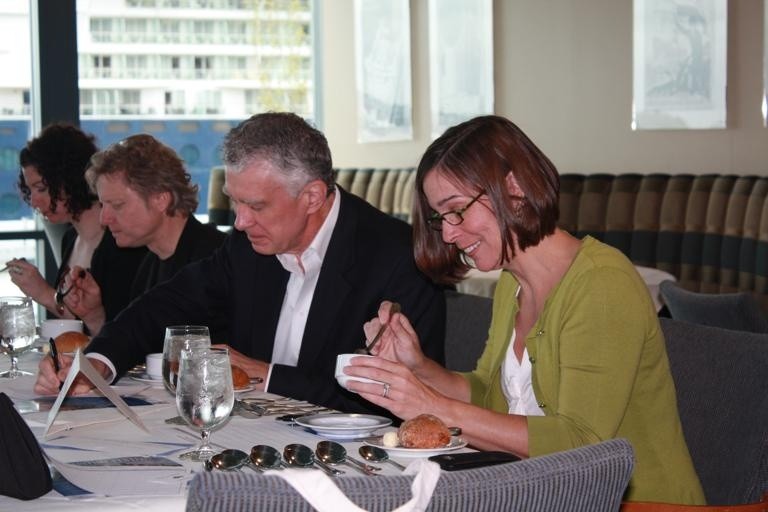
[202,165,768,323]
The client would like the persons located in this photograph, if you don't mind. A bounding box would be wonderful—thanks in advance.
[0,123,146,341]
[341,116,713,506]
[35,112,449,427]
[54,131,239,346]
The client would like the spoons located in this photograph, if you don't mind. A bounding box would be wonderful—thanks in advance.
[236,391,321,417]
[55,269,87,315]
[202,441,407,482]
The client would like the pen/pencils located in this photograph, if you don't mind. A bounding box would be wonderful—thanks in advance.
[49,337,63,390]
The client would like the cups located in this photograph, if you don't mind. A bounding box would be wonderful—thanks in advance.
[41,319,82,343]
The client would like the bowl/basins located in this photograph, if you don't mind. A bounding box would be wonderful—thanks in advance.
[146,353,163,379]
[333,353,384,393]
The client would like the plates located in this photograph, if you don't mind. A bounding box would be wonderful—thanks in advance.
[297,412,392,437]
[130,376,164,389]
[370,435,467,456]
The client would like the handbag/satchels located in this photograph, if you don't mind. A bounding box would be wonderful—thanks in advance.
[0,392,53,501]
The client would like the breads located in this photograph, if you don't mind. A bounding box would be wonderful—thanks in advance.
[398,412,451,450]
[229,363,251,391]
[50,330,91,354]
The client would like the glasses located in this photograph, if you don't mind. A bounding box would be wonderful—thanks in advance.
[425,191,485,231]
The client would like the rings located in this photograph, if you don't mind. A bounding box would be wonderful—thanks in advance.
[380,382,390,396]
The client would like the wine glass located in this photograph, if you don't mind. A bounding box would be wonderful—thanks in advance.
[164,327,211,425]
[0,298,37,380]
[175,348,236,461]
